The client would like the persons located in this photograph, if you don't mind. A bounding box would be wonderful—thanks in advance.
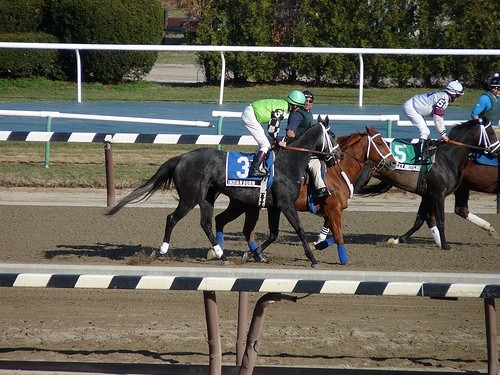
[285,91,337,197]
[471,78,500,128]
[403,80,466,162]
[241,91,306,178]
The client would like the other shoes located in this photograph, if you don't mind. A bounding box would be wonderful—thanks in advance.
[313,187,331,200]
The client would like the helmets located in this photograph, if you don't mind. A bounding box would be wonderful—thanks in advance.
[444,80,464,95]
[302,90,314,100]
[490,73,500,86]
[287,90,306,106]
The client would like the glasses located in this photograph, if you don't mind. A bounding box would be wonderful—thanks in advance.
[491,86,500,91]
[305,99,313,103]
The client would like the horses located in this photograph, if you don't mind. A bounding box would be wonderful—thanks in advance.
[101,114,344,269]
[215,125,397,266]
[383,117,500,250]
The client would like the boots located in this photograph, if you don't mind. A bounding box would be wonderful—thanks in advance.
[248,150,268,178]
[416,138,429,163]
